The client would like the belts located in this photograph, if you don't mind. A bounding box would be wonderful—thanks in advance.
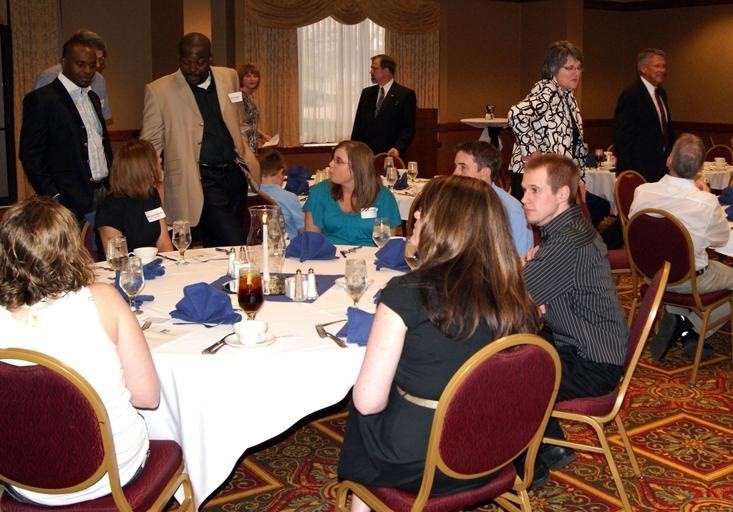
[655,145,671,151]
[199,163,235,170]
[395,383,438,412]
[90,179,111,188]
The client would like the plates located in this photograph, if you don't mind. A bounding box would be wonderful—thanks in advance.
[223,334,277,347]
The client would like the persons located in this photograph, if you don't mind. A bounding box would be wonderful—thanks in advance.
[338,176,546,512]
[1,196,160,507]
[508,40,586,203]
[613,48,677,183]
[629,133,733,361]
[137,33,261,246]
[237,63,271,159]
[95,141,175,260]
[17,33,115,220]
[349,54,417,156]
[452,143,536,261]
[512,155,630,489]
[32,30,114,129]
[259,153,306,240]
[301,140,403,247]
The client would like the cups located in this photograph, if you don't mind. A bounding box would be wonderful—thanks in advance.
[133,247,158,261]
[232,317,270,344]
[712,156,729,171]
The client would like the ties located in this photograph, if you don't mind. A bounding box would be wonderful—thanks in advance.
[654,88,669,143]
[374,86,385,121]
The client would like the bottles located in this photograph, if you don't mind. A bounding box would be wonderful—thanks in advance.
[306,268,318,300]
[238,246,247,265]
[295,268,304,300]
[229,247,235,279]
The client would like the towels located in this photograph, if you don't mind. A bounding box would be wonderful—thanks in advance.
[142,258,166,277]
[169,281,241,327]
[286,167,313,180]
[376,237,410,271]
[115,271,155,309]
[336,307,376,345]
[695,264,709,278]
[394,172,409,189]
[286,176,309,194]
[285,231,337,262]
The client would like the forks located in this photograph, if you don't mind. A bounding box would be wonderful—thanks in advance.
[213,247,231,256]
[172,320,225,329]
[139,320,150,332]
[315,324,346,348]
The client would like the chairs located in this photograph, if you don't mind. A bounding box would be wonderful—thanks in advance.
[335,334,561,512]
[0,347,196,512]
[540,261,670,512]
[613,169,647,336]
[625,208,732,383]
[704,144,732,166]
[372,152,405,178]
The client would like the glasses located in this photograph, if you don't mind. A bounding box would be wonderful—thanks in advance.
[559,65,583,72]
[96,57,104,64]
[327,157,351,165]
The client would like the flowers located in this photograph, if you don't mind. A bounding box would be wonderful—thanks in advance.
[481,103,495,114]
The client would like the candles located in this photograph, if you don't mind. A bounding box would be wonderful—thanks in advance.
[262,214,270,280]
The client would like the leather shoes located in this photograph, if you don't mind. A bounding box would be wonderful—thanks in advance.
[538,437,576,468]
[515,453,550,492]
[683,326,715,362]
[648,314,682,361]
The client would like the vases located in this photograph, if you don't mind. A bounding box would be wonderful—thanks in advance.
[484,112,493,120]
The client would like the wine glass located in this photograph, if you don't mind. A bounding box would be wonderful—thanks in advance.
[383,158,393,176]
[117,257,144,319]
[107,236,126,278]
[238,266,263,321]
[386,167,396,191]
[594,149,604,168]
[407,162,417,185]
[170,221,192,263]
[344,259,370,306]
